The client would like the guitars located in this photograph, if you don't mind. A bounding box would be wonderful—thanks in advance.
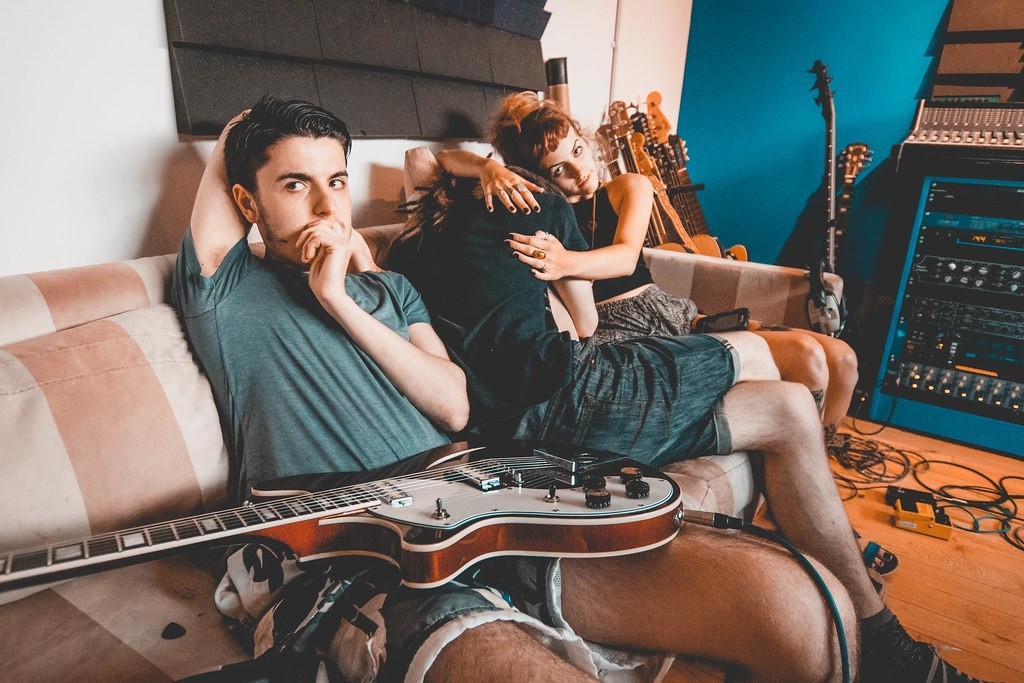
[595,90,752,259]
[803,59,877,337]
[0,435,686,593]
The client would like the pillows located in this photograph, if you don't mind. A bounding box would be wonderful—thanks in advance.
[0,304,232,607]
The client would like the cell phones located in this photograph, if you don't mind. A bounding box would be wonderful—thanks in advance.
[695,307,750,335]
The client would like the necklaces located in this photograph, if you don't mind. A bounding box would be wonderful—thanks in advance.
[566,192,599,231]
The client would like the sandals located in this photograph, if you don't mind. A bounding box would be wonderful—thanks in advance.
[853,529,898,575]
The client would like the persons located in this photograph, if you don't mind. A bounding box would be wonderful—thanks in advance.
[379,167,987,683]
[430,87,860,453]
[170,94,856,683]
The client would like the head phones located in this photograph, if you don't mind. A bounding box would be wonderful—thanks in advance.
[806,259,848,339]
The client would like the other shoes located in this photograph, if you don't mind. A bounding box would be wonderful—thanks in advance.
[905,642,984,683]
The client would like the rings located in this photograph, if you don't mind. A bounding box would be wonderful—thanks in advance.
[532,248,546,259]
[544,231,548,240]
[540,261,548,272]
[506,187,514,198]
[495,189,506,195]
[517,182,525,192]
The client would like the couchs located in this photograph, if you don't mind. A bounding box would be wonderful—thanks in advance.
[0,223,845,683]
